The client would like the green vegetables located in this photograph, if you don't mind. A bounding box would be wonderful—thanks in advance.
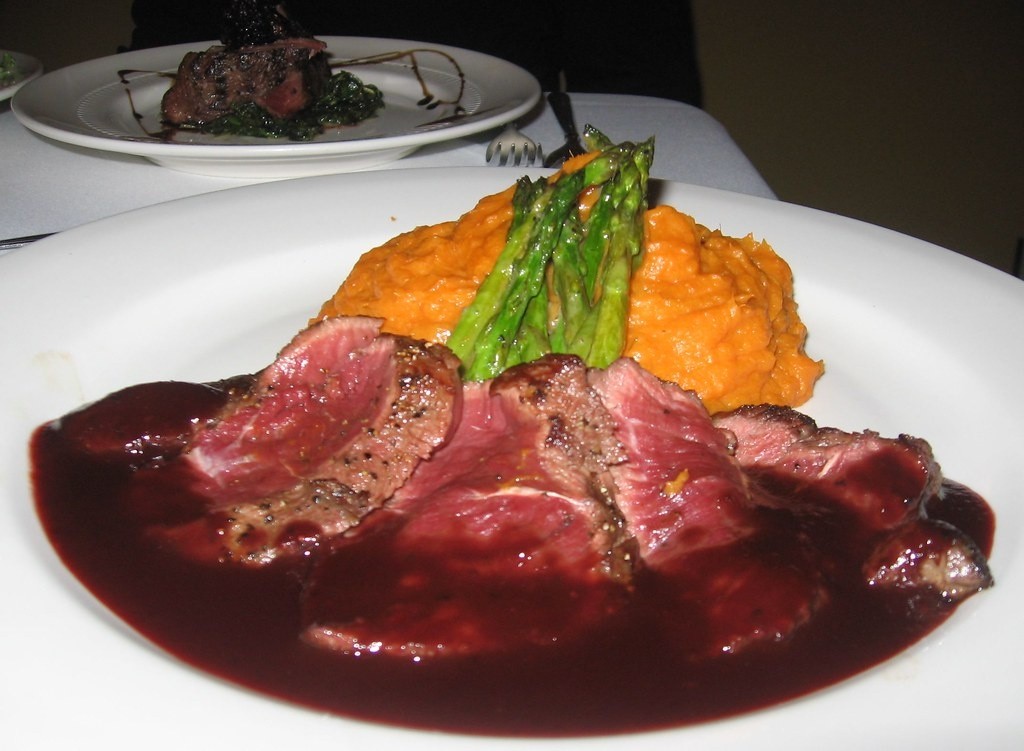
[160,71,384,141]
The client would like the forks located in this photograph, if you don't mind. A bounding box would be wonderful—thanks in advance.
[486,121,544,168]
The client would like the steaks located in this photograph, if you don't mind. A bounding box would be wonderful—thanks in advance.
[160,0,333,125]
[135,314,997,663]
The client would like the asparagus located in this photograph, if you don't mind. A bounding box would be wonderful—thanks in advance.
[443,122,658,382]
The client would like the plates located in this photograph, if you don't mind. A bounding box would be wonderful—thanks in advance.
[0,166,1024,751]
[0,50,42,100]
[10,36,542,179]
